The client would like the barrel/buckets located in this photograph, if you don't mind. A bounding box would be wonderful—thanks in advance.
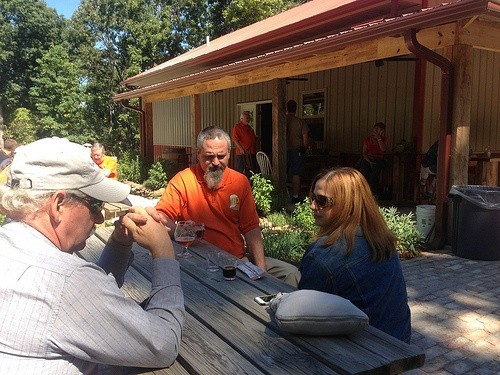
[415,205,436,238]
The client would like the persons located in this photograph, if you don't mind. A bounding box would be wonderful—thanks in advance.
[286,99,309,205]
[154,125,301,288]
[0,129,185,375]
[359,122,388,194]
[297,167,411,344]
[231,111,257,173]
[416,140,438,205]
[90,143,132,206]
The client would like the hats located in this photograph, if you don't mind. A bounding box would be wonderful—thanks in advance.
[5,137,131,203]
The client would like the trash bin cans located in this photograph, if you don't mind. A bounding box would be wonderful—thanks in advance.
[448,184,500,261]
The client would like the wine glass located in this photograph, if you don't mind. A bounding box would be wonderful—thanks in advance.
[174,221,196,258]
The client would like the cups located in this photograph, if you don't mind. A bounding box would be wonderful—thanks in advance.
[223,257,237,280]
[207,251,220,272]
[197,224,205,239]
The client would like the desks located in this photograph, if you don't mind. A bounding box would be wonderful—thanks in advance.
[73,225,426,375]
[375,153,425,206]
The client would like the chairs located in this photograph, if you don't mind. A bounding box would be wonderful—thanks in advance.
[255,151,273,176]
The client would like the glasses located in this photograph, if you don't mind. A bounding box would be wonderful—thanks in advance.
[72,193,105,215]
[309,192,336,208]
